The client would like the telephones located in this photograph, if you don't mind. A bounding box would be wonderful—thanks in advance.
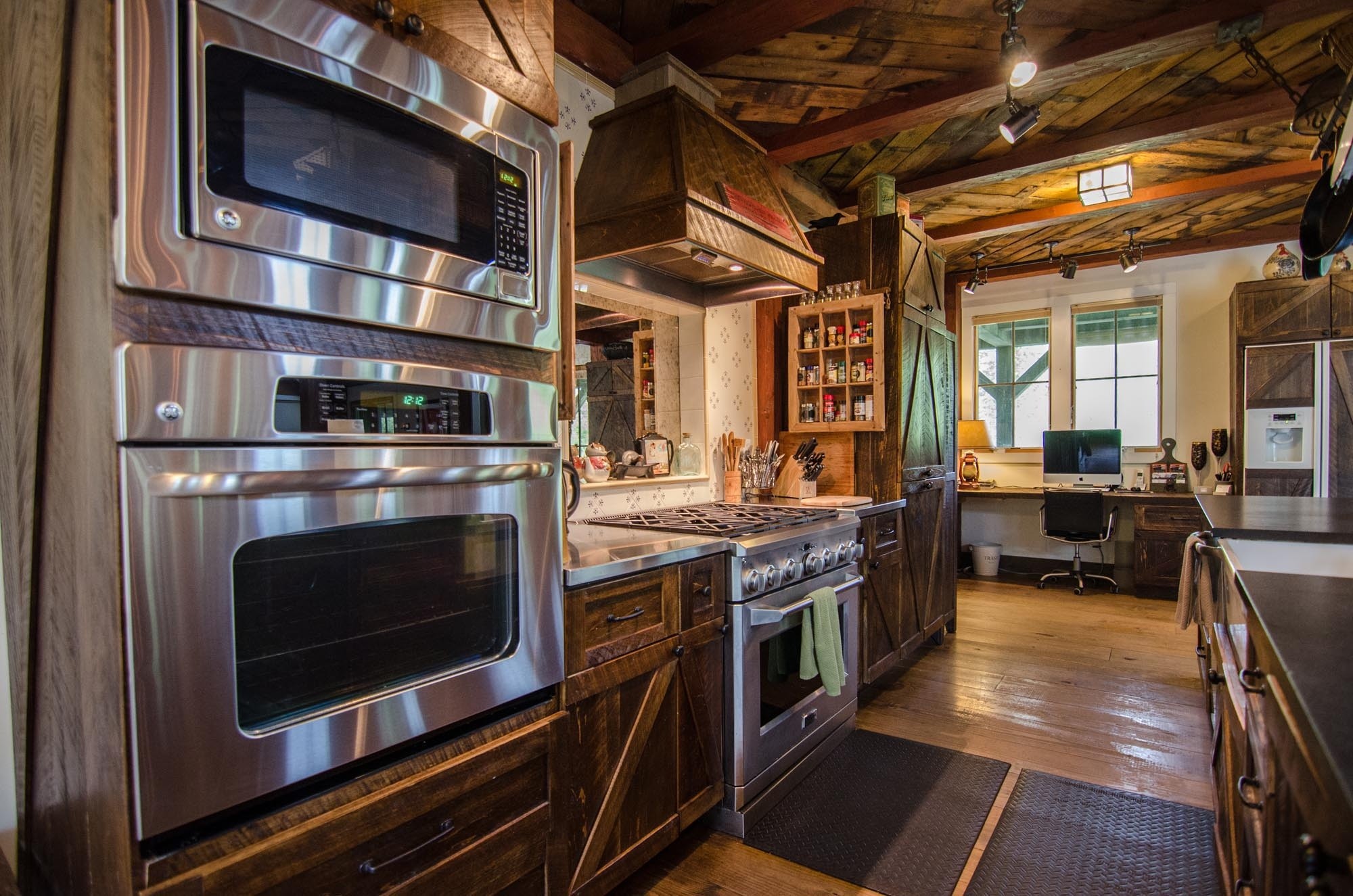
[1134,469,1148,491]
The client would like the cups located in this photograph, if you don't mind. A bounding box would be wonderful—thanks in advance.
[723,470,741,503]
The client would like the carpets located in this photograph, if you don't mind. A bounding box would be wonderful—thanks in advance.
[963,767,1227,896]
[742,728,1011,896]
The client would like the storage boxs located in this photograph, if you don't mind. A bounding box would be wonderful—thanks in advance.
[910,214,925,231]
[856,173,896,220]
[895,191,910,218]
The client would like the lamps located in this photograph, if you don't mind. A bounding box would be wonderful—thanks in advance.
[1119,227,1144,273]
[1043,241,1078,279]
[964,252,988,295]
[958,420,995,489]
[1290,64,1353,136]
[993,0,1037,88]
[1077,161,1132,207]
[999,79,1041,145]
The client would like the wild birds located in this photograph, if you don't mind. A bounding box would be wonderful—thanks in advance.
[808,213,846,230]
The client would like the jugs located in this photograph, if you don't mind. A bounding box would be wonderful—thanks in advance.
[580,442,611,482]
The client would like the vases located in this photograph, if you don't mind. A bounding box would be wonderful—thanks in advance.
[1262,244,1301,279]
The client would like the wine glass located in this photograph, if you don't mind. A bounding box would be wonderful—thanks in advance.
[1191,441,1207,487]
[1211,428,1228,474]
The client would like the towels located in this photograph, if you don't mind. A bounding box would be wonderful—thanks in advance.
[1173,536,1215,630]
[800,586,847,697]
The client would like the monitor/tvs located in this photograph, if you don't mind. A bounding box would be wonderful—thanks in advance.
[1043,429,1121,493]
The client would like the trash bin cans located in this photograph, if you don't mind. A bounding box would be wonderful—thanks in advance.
[969,542,1002,576]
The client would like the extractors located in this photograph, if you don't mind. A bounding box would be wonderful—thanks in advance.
[575,57,825,310]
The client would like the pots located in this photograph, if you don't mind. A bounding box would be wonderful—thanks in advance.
[1297,123,1353,259]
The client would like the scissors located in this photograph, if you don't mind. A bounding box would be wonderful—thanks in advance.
[1223,463,1232,481]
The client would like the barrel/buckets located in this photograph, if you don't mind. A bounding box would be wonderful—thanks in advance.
[972,541,1002,576]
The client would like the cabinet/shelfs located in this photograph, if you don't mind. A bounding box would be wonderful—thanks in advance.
[1135,270,1353,896]
[0,0,957,896]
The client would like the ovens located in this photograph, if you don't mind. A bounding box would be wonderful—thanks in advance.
[114,340,568,860]
[704,560,860,838]
[110,0,563,355]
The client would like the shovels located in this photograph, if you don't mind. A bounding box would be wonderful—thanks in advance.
[728,432,734,471]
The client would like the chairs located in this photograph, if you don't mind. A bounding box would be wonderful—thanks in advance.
[1037,488,1120,596]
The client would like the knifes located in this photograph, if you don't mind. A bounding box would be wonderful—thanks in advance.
[793,437,825,481]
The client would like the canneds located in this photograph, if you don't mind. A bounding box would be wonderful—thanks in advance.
[798,320,873,386]
[824,394,874,422]
[800,403,819,423]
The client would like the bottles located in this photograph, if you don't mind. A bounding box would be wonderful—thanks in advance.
[649,349,654,367]
[1328,251,1351,274]
[643,352,649,368]
[799,281,861,306]
[1262,244,1301,279]
[643,410,651,430]
[674,432,702,476]
[643,380,649,399]
[650,409,655,429]
[648,382,655,398]
[798,321,874,421]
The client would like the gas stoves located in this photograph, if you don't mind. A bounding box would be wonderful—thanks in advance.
[588,501,864,602]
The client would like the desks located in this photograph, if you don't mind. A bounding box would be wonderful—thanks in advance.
[957,487,1197,600]
